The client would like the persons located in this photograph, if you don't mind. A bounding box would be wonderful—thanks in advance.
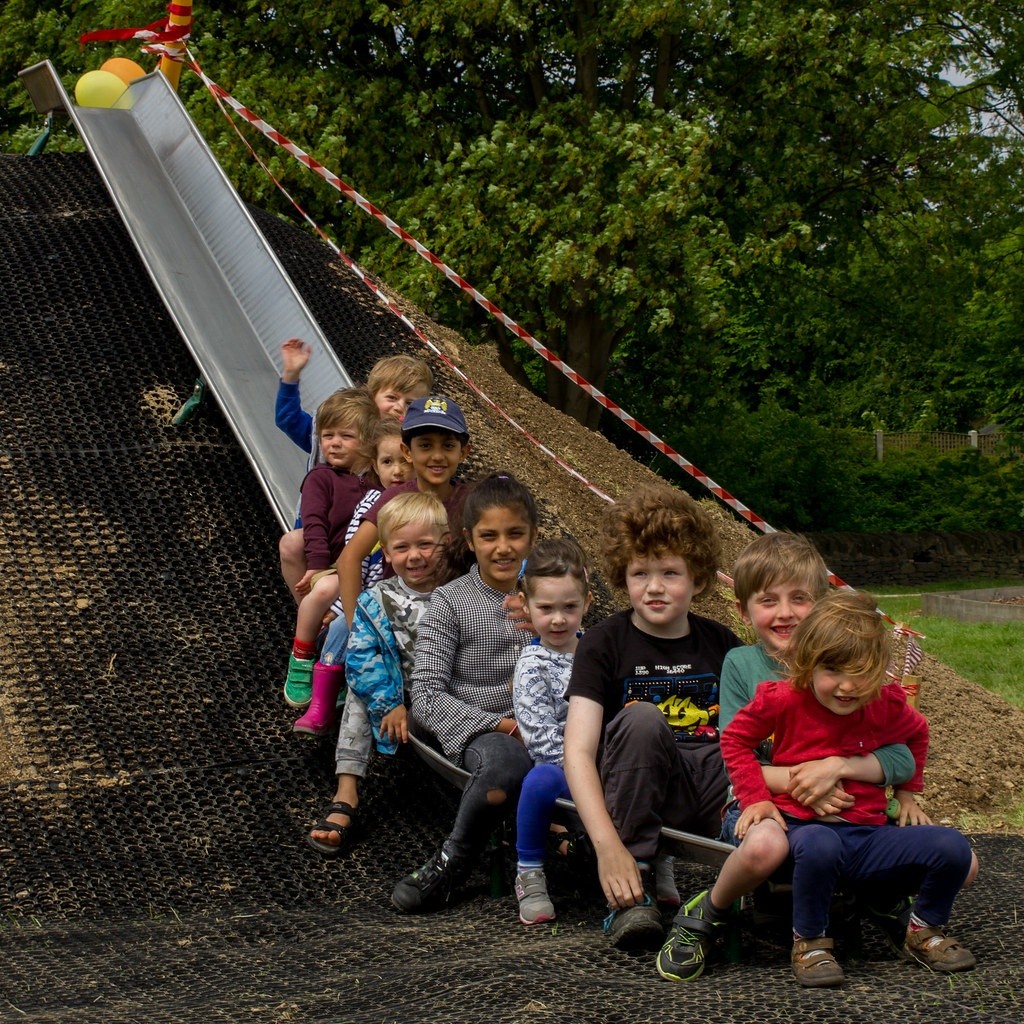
[563,489,773,953]
[278,338,978,986]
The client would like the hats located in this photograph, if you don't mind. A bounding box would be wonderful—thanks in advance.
[400,395,470,443]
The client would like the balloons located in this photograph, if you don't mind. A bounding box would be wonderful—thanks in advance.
[101,57,148,87]
[74,70,133,110]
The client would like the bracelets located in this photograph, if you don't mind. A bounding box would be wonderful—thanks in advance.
[509,724,518,737]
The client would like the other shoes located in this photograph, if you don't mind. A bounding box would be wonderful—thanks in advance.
[316,623,329,651]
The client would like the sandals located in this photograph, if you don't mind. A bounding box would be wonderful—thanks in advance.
[791,938,844,987]
[308,802,361,854]
[903,926,976,972]
[548,830,572,861]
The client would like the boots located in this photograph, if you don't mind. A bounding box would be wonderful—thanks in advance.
[294,661,344,737]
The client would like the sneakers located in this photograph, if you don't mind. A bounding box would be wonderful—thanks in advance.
[567,834,596,876]
[391,849,472,914]
[868,895,916,947]
[604,870,666,952]
[650,854,681,906]
[514,869,557,924]
[284,652,317,708]
[656,890,731,982]
[752,885,785,927]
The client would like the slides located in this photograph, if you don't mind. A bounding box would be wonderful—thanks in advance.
[16,57,738,851]
